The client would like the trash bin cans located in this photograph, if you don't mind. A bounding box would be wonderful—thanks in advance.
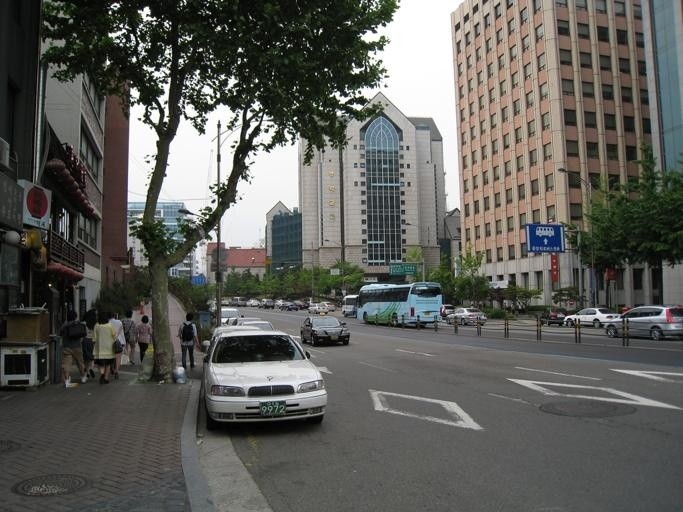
[197,312,209,326]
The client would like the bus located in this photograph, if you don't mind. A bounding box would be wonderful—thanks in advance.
[354,281,443,328]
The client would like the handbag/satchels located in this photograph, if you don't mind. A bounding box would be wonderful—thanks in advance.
[125,331,130,341]
[114,340,123,353]
[67,324,86,338]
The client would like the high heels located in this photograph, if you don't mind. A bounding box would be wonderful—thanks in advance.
[115,372,119,379]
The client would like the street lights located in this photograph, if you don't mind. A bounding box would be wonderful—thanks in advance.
[175,117,275,326]
[403,220,431,282]
[556,166,596,306]
[323,239,346,287]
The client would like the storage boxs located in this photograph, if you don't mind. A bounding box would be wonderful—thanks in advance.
[6,312,50,344]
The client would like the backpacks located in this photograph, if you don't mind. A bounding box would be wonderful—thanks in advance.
[182,322,194,340]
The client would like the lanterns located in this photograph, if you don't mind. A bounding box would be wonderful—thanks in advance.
[46,158,94,218]
[26,185,48,219]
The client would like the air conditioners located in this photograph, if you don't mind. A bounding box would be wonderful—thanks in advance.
[0,137,11,172]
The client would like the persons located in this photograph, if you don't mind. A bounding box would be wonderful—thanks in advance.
[58,308,153,388]
[178,313,199,369]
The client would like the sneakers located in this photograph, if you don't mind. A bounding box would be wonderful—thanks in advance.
[63,376,71,387]
[89,369,95,378]
[81,376,88,383]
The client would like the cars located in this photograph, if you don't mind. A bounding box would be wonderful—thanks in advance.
[446,308,487,325]
[198,294,334,429]
[563,307,621,328]
[539,307,570,327]
[300,314,351,346]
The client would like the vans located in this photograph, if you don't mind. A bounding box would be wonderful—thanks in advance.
[338,293,359,319]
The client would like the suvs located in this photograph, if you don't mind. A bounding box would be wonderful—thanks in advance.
[443,303,455,317]
[602,305,683,341]
